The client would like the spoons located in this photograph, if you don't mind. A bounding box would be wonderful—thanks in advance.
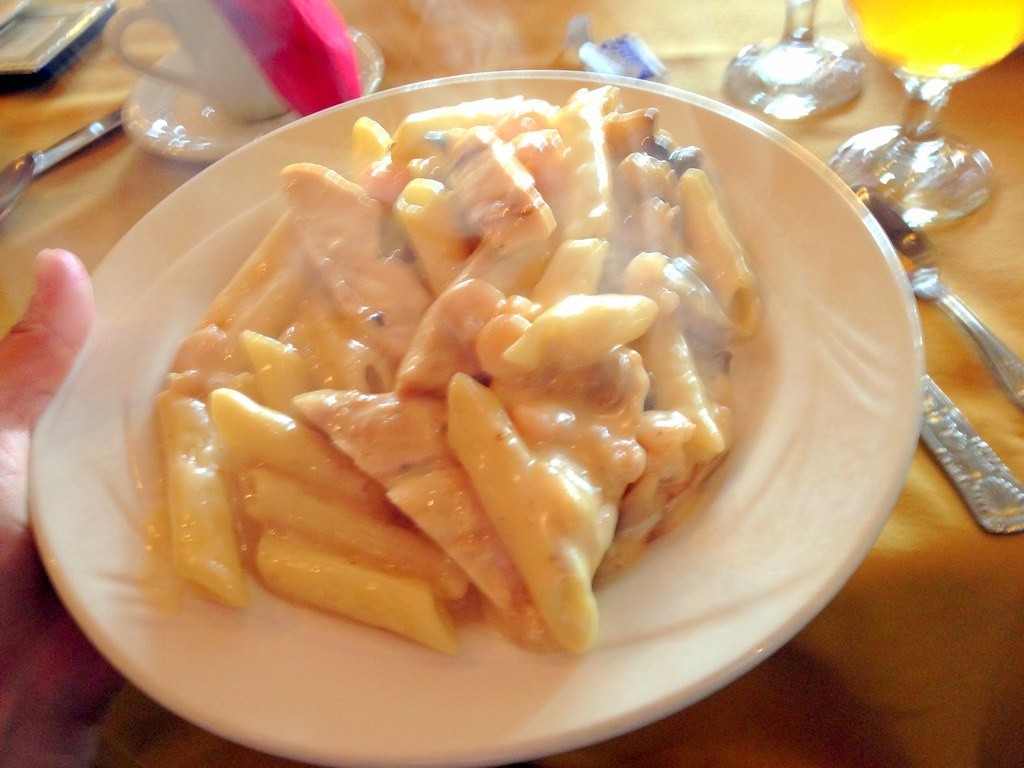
[849,184,1023,411]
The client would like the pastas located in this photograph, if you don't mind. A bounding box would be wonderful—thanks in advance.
[154,98,752,656]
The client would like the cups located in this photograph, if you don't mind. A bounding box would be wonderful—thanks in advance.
[103,0,294,122]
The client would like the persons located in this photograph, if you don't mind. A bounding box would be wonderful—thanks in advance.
[1,244,168,768]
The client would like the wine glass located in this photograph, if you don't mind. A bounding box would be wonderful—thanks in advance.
[725,0,863,120]
[827,0,1024,227]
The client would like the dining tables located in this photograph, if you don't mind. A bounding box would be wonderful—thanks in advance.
[0,0,1024,768]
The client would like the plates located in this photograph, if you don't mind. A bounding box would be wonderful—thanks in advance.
[0,0,115,76]
[122,27,384,164]
[28,70,925,768]
